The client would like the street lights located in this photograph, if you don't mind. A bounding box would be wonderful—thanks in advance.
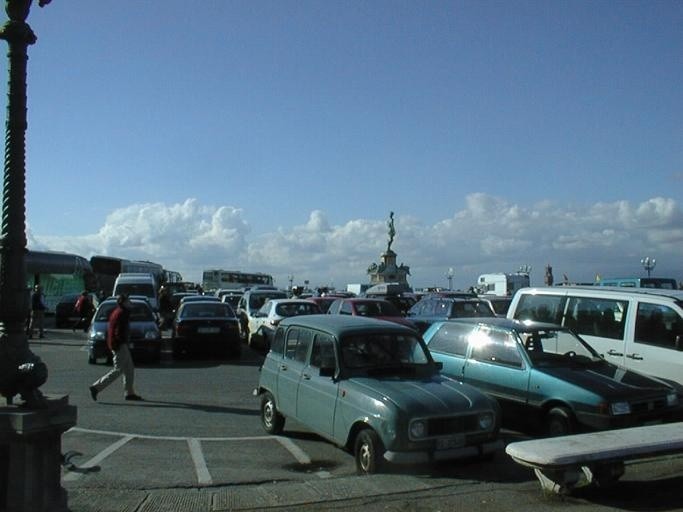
[640,256,656,278]
[518,264,532,276]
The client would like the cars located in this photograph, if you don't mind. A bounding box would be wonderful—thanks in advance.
[81,300,163,364]
[412,317,682,438]
[252,314,503,477]
[55,293,101,328]
[88,272,511,355]
[169,301,243,361]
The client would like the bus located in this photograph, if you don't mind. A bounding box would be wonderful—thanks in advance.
[202,268,277,290]
[88,256,166,304]
[593,278,679,291]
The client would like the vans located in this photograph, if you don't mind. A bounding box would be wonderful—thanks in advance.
[505,286,682,387]
[476,273,531,300]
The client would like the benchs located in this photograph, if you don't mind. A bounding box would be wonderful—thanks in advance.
[504,421,683,493]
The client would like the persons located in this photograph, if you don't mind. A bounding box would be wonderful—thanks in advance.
[156,287,170,330]
[72,289,90,334]
[88,291,145,402]
[24,284,51,339]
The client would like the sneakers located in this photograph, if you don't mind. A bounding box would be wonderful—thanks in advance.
[89,386,98,401]
[125,394,144,401]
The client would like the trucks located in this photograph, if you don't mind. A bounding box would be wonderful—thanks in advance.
[24,249,95,317]
